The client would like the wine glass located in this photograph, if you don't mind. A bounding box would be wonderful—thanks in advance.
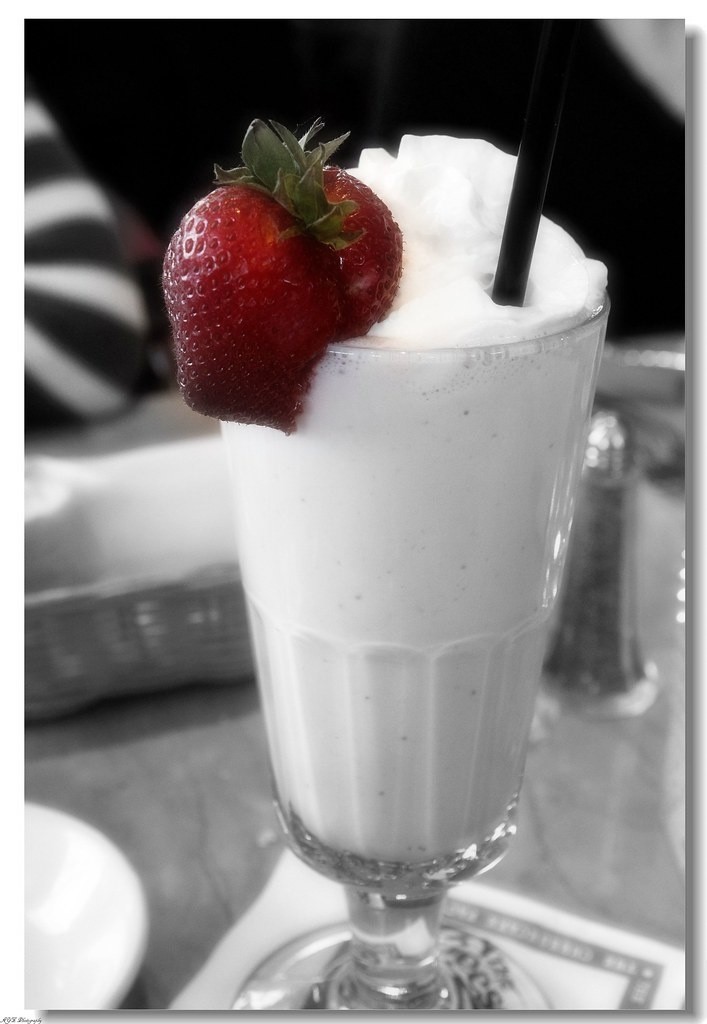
[222,267,611,1010]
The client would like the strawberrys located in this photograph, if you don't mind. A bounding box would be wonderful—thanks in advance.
[161,118,404,433]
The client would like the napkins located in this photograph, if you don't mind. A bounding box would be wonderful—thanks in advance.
[174,842,686,1011]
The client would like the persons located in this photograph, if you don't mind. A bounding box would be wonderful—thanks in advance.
[25,79,152,431]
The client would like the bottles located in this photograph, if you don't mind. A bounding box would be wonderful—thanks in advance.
[532,410,659,718]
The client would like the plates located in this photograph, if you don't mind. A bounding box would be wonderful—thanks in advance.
[25,802,149,1010]
